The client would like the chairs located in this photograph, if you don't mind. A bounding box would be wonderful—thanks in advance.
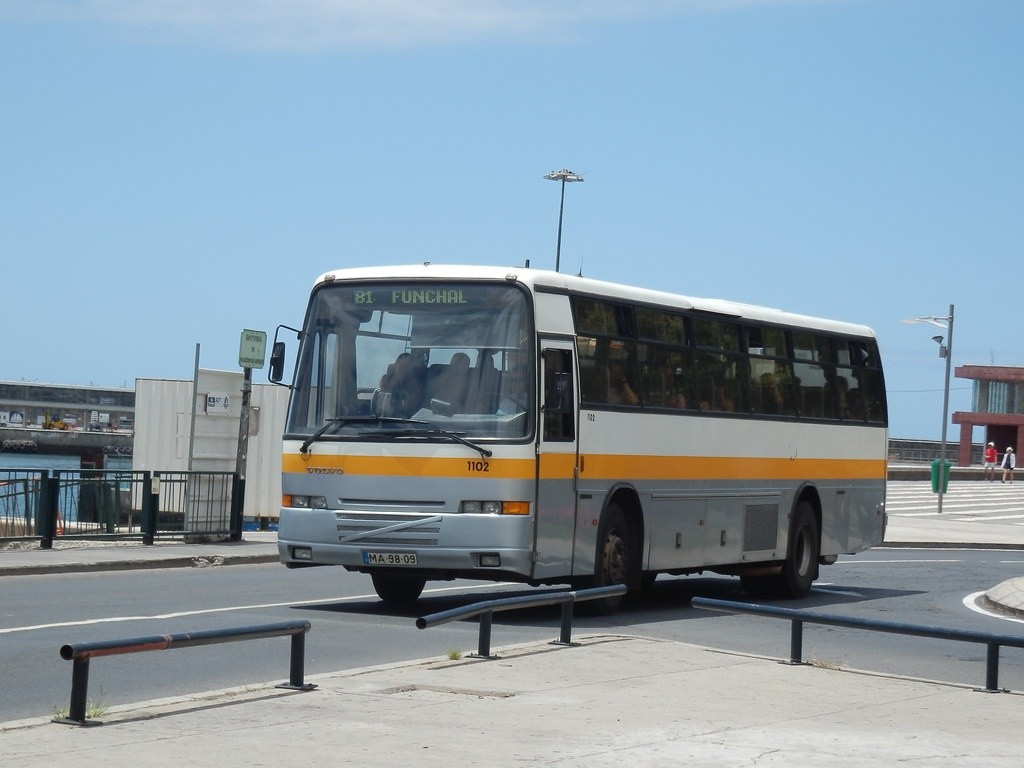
[422,365,850,442]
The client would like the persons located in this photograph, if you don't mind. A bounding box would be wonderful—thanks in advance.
[984,442,997,483]
[380,352,853,420]
[1001,447,1015,484]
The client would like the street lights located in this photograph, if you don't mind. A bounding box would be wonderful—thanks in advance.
[898,302,955,515]
[541,169,587,275]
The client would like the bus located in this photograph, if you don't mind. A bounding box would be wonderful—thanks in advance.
[268,262,890,619]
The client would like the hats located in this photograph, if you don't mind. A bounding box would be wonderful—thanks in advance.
[988,442,994,447]
[1006,447,1013,453]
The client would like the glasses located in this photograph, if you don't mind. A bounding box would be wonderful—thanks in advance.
[511,378,527,384]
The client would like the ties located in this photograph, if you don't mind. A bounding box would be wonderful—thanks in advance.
[515,395,523,413]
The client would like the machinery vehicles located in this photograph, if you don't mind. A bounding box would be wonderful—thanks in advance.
[42,409,68,430]
